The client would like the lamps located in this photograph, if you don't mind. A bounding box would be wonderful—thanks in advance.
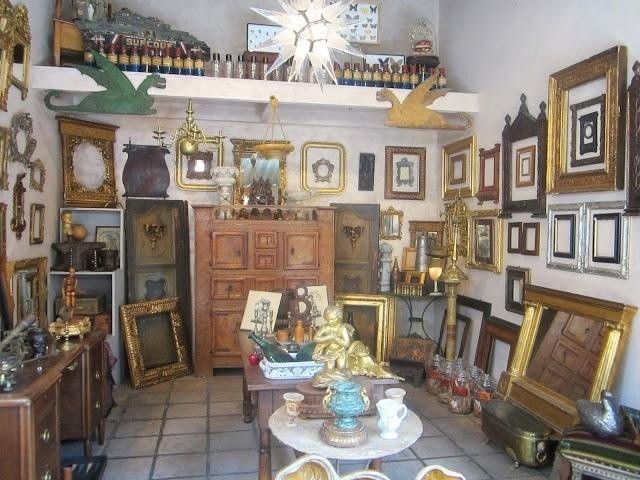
[249,0,368,93]
[153,100,227,160]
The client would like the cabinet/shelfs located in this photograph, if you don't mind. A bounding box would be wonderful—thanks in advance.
[193,204,338,377]
[0,329,107,480]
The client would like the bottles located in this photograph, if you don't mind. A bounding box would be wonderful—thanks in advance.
[428,353,497,418]
[391,257,401,282]
[334,61,447,88]
[211,53,316,83]
[414,235,429,271]
[247,331,295,362]
[85,34,203,76]
[294,320,304,342]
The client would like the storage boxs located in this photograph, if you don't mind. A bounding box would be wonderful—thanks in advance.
[54,295,106,316]
[56,312,111,333]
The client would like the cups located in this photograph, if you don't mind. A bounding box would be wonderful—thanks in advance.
[277,329,289,342]
[385,388,405,402]
[376,399,407,439]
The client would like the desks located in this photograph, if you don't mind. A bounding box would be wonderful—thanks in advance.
[381,291,449,357]
[236,328,424,480]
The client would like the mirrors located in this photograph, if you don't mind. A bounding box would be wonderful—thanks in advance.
[496,284,637,436]
[6,256,48,332]
[0,0,31,112]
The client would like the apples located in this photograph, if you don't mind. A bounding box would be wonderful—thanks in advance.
[248,351,262,366]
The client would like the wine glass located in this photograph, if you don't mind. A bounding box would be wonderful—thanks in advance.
[283,392,304,427]
[429,267,443,296]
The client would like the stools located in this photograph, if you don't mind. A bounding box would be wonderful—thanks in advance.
[557,403,640,480]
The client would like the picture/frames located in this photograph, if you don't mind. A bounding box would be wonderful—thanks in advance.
[49,204,126,395]
[175,136,224,191]
[435,265,532,375]
[0,113,46,244]
[231,138,289,214]
[335,293,397,362]
[363,53,405,74]
[119,297,192,390]
[300,141,346,195]
[56,116,120,208]
[95,226,120,269]
